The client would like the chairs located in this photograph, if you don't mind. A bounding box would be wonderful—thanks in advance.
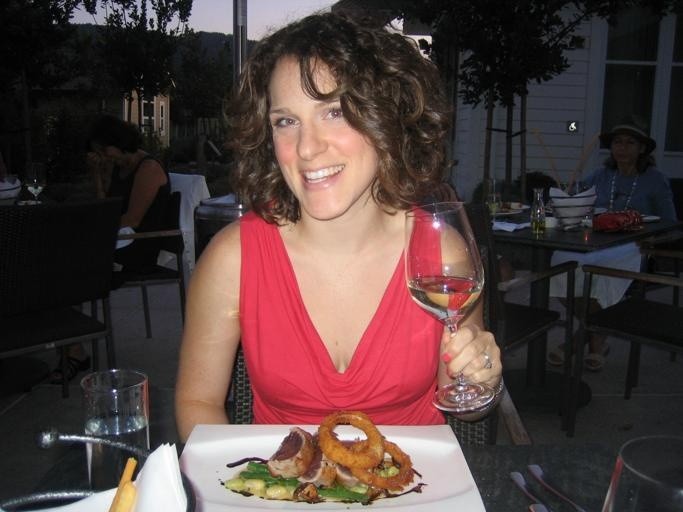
[2,197,124,398]
[624,178,681,363]
[465,202,577,445]
[113,191,185,340]
[517,173,563,204]
[574,246,683,399]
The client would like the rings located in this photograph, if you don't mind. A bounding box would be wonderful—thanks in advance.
[483,351,494,370]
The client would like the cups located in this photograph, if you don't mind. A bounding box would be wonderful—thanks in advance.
[601,436,683,511]
[78,369,151,491]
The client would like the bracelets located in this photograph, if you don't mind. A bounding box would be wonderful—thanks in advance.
[494,377,504,397]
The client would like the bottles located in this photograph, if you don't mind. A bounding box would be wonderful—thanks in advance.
[530,188,546,233]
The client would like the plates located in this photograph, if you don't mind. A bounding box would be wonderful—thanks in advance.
[178,424,486,512]
[641,214,662,224]
[497,203,530,216]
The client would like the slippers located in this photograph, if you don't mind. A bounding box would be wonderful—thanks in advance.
[49,356,90,384]
[584,345,610,371]
[547,344,577,365]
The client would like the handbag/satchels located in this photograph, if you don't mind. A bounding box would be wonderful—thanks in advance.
[593,209,640,231]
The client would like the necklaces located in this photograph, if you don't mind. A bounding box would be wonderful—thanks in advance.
[609,169,638,214]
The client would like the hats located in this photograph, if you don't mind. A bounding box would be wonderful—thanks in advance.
[598,114,655,156]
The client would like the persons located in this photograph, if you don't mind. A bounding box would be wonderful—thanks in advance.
[546,110,677,371]
[49,113,171,384]
[173,9,505,449]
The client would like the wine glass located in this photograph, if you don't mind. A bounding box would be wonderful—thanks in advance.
[486,192,502,223]
[403,201,498,413]
[25,162,44,205]
